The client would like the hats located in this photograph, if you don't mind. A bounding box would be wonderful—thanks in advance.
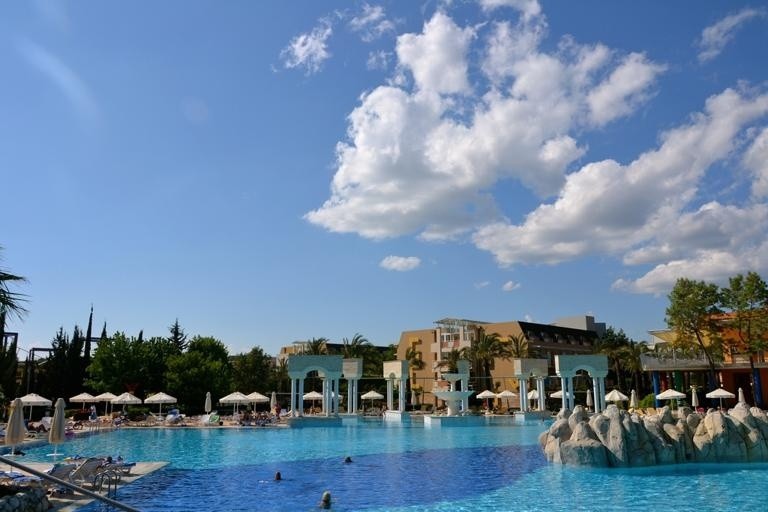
[322,491,331,500]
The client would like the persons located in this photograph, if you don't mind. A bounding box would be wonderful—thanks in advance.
[274,402,281,421]
[276,472,281,480]
[321,492,331,509]
[90,403,97,420]
[238,411,271,426]
[25,420,48,433]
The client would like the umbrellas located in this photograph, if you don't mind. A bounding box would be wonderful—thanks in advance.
[476,387,746,410]
[411,391,416,412]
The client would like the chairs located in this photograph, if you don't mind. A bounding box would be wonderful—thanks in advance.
[23,418,40,438]
[0,460,137,498]
